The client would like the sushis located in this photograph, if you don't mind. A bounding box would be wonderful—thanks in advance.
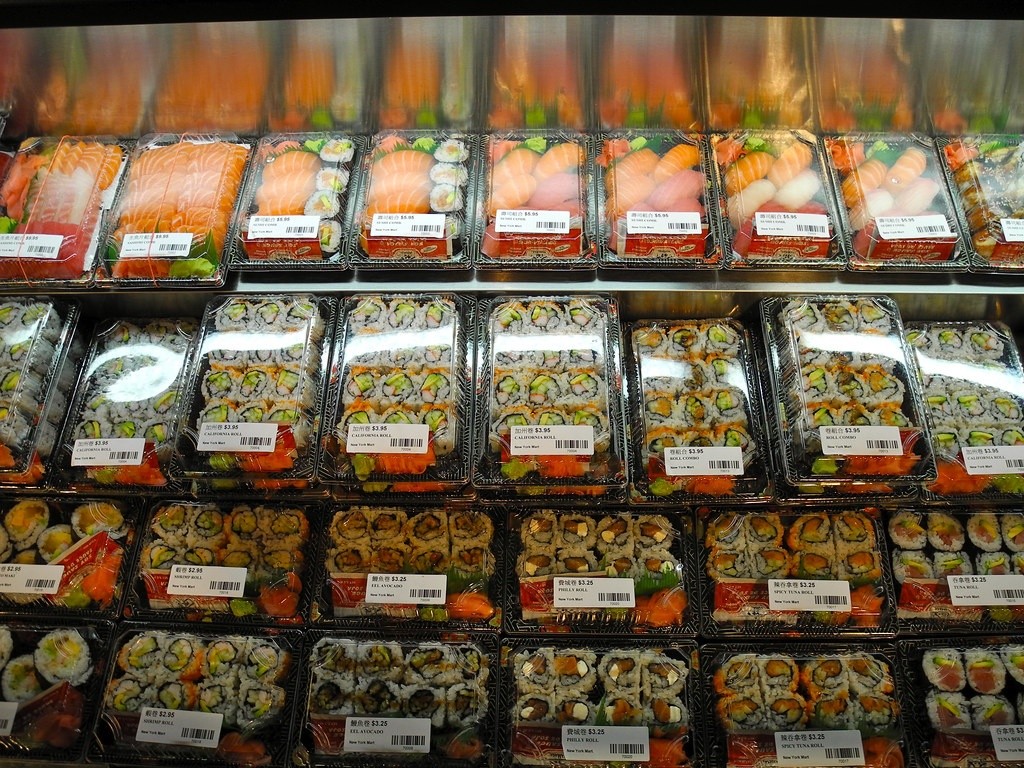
[22,141,1024,258]
[0,298,1024,736]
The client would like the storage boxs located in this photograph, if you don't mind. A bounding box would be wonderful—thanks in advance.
[0,127,1024,768]
